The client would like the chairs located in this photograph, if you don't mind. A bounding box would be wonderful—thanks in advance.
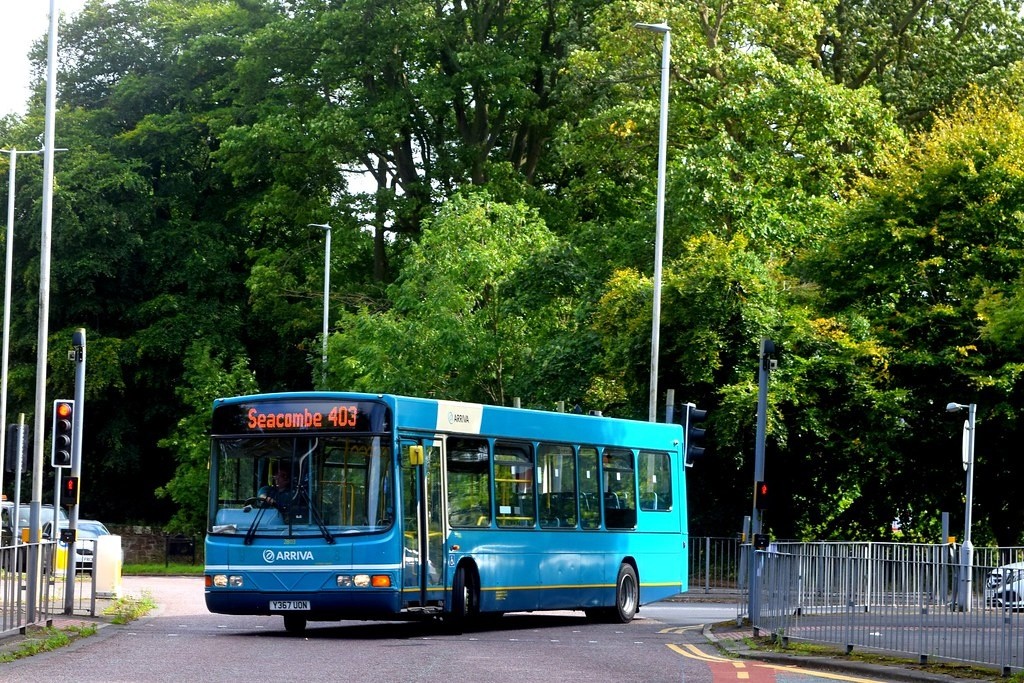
[449,491,657,528]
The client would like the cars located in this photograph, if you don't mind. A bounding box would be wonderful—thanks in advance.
[2,499,69,571]
[982,560,1024,614]
[39,518,111,579]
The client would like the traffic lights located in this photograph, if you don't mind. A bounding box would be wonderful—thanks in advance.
[51,398,77,469]
[681,402,709,468]
[758,479,772,506]
[62,476,79,508]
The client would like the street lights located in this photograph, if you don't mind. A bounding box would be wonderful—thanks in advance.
[636,19,674,422]
[945,401,977,616]
[1,145,71,459]
[307,219,334,392]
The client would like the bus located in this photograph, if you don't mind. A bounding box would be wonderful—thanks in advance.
[204,393,690,636]
